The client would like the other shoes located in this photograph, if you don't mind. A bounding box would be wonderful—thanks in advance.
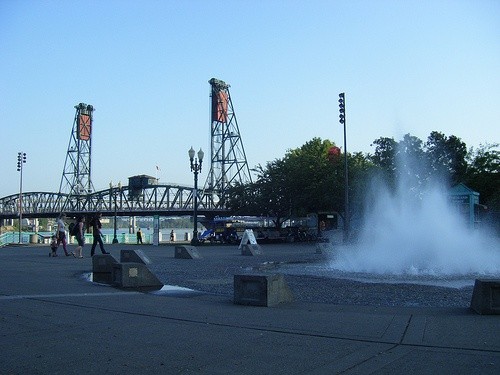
[49,253,51,256]
[71,251,76,257]
[104,252,109,254]
[66,253,71,256]
[79,256,83,258]
[53,255,55,257]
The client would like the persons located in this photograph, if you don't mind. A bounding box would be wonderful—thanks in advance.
[71,215,85,259]
[170,230,174,241]
[137,228,143,245]
[48,211,71,257]
[91,210,111,256]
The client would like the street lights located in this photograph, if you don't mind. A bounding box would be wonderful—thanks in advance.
[108,180,121,245]
[16,151,27,245]
[187,146,205,246]
[339,92,350,246]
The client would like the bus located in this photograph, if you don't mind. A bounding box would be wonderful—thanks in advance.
[210,218,289,242]
